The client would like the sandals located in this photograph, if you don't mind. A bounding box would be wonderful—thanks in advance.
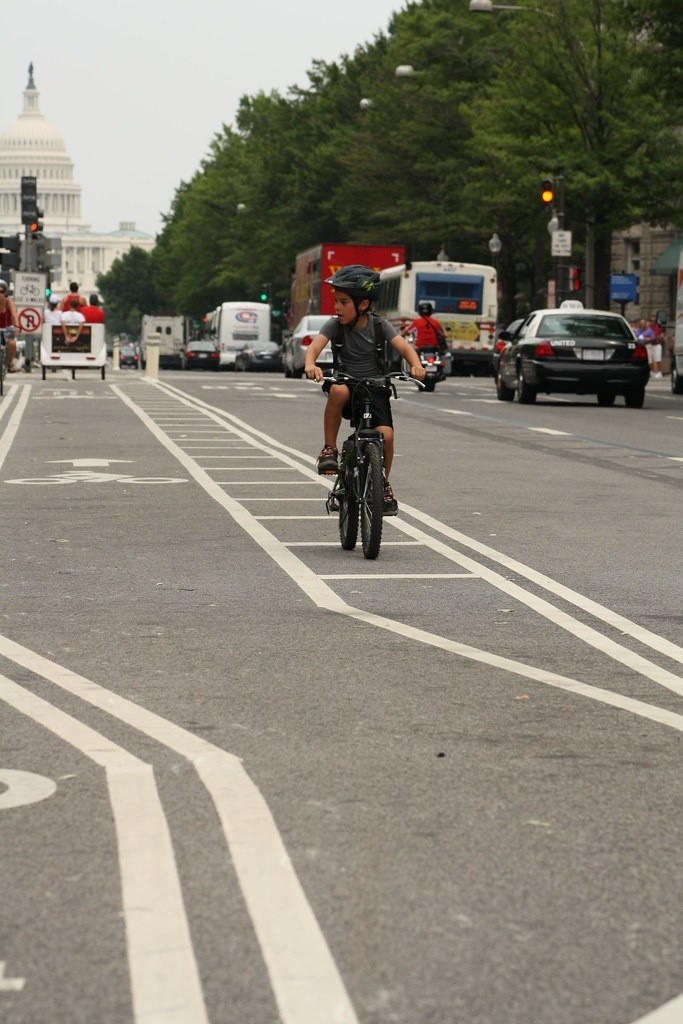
[7,364,16,373]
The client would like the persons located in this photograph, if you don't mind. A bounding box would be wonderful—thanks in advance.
[60,282,89,311]
[42,294,63,322]
[59,298,86,343]
[630,318,666,378]
[304,265,427,516]
[400,301,448,382]
[0,280,22,373]
[80,294,105,322]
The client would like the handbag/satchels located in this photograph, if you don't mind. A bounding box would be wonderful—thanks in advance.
[438,334,448,351]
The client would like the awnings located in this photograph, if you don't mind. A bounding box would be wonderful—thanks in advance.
[648,237,683,276]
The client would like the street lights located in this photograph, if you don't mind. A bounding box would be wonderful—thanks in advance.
[488,233,503,270]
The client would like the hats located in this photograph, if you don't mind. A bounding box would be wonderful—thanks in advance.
[0,279,8,290]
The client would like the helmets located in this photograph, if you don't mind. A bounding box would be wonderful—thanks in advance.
[323,265,382,291]
[419,303,433,316]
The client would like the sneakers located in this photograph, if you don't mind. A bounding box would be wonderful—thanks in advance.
[382,483,398,517]
[316,446,340,475]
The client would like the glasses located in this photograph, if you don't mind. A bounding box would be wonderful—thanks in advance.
[0,290,5,293]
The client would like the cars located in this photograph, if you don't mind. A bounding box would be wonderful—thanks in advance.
[491,318,525,384]
[281,314,343,380]
[495,299,651,409]
[179,340,219,371]
[233,339,281,373]
[118,349,139,370]
[14,333,38,354]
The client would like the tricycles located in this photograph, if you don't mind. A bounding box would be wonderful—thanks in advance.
[39,322,106,381]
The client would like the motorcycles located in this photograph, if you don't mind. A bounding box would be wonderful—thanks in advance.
[398,332,453,392]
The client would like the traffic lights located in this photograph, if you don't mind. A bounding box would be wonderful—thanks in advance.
[0,236,20,269]
[542,179,553,213]
[45,272,51,299]
[261,285,268,303]
[34,207,44,231]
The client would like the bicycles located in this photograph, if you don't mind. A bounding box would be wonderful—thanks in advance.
[305,368,428,558]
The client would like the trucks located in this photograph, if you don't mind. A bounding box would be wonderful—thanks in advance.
[140,315,185,370]
[669,250,683,394]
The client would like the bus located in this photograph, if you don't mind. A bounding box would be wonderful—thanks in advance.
[190,306,222,340]
[218,301,271,369]
[281,243,405,336]
[371,260,498,377]
